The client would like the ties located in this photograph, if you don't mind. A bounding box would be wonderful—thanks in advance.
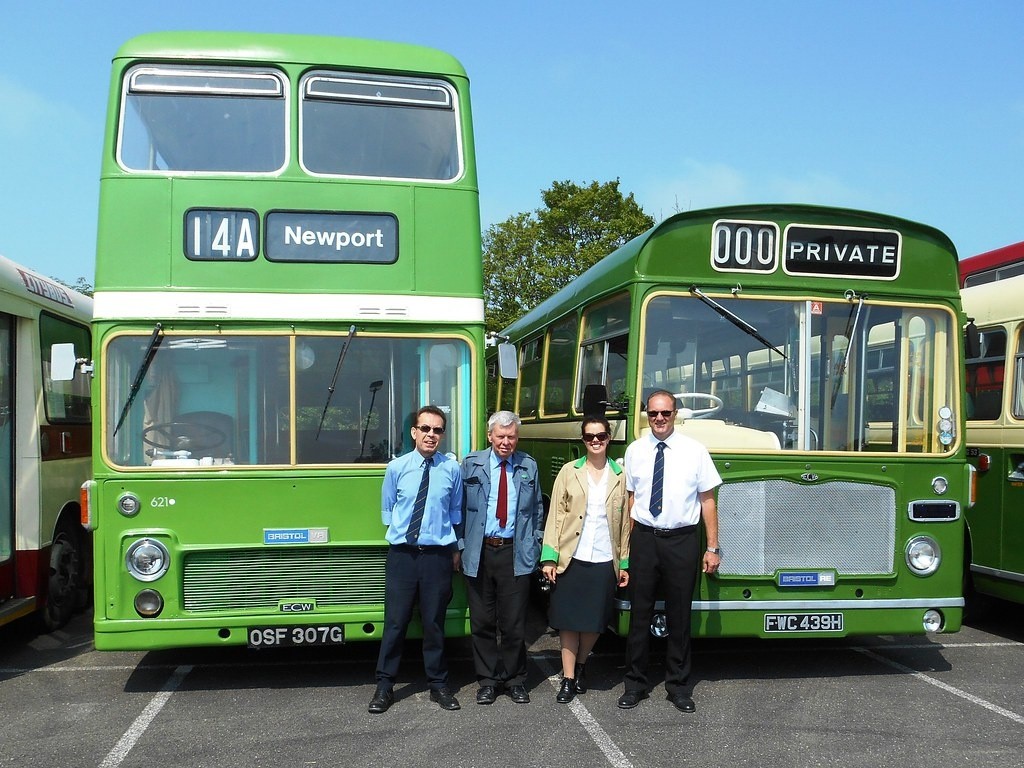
[405,459,433,544]
[648,442,667,518]
[495,460,508,527]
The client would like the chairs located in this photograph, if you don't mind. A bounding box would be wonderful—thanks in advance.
[166,410,233,461]
[635,387,684,414]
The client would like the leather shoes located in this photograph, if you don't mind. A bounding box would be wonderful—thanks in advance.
[574,663,588,694]
[618,690,650,709]
[666,693,695,713]
[503,685,530,703]
[430,687,460,710]
[369,687,394,712]
[476,686,499,703]
[556,677,576,702]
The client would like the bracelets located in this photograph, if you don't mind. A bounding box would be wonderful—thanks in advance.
[706,547,721,554]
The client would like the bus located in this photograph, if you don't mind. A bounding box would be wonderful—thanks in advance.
[482,204,981,638]
[51,30,517,650]
[0,254,132,630]
[644,241,1024,606]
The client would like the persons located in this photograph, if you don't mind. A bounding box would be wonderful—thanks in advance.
[540,416,631,704]
[452,410,544,705]
[617,391,724,712]
[366,404,463,713]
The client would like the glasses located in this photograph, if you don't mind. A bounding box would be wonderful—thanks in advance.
[414,424,444,434]
[647,410,674,417]
[583,432,608,442]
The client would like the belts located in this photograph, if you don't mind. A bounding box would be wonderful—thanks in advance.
[634,521,702,538]
[390,543,449,554]
[483,537,513,547]
